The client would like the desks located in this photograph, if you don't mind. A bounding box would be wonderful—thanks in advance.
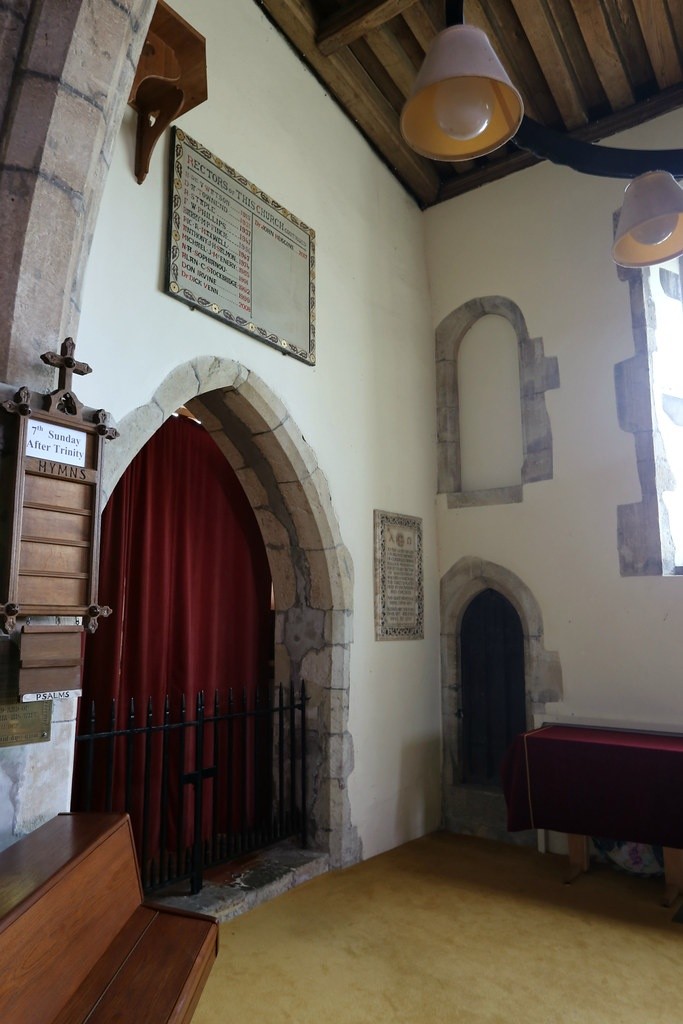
[498,725,683,908]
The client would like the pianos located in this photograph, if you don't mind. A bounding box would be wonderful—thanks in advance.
[1,811,219,1023]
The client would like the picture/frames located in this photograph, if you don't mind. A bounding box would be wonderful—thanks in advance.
[373,509,425,641]
[162,125,317,369]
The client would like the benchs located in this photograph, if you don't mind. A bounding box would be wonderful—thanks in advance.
[1,813,221,1024]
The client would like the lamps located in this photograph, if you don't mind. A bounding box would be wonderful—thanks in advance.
[610,170,683,269]
[399,24,526,165]
